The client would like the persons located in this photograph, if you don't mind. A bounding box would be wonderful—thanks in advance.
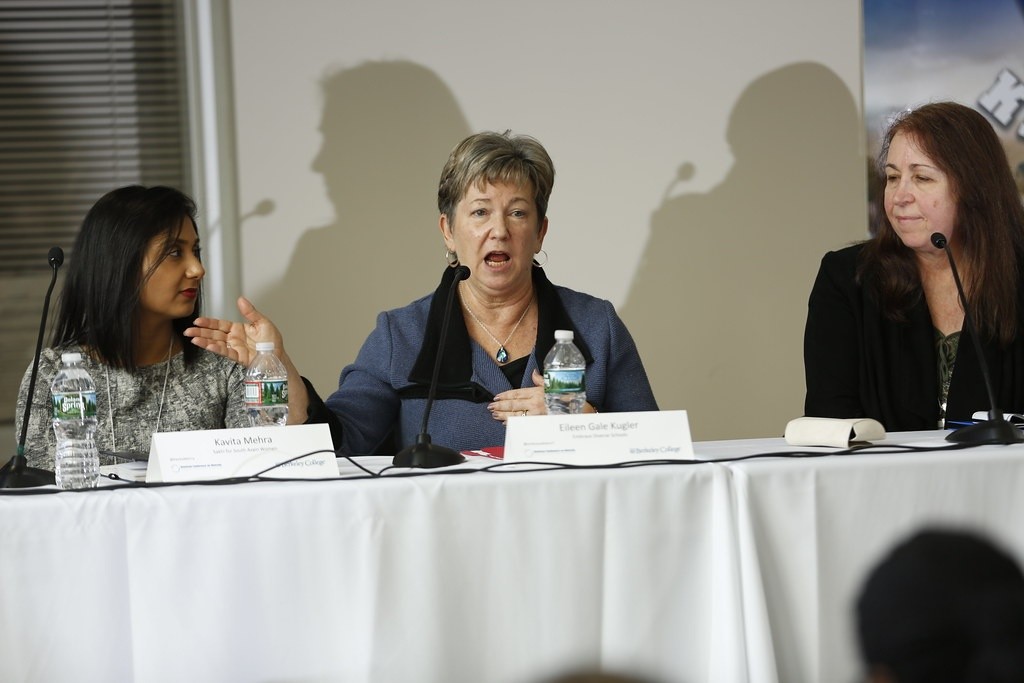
[15,185,252,472]
[854,527,1024,683]
[804,101,1024,432]
[182,129,661,457]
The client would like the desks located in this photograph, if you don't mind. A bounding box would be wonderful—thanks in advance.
[0,428,1024,683]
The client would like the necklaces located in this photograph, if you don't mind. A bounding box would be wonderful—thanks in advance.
[458,280,538,363]
[103,334,175,465]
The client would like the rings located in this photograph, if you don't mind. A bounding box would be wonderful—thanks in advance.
[227,342,231,349]
[522,410,527,417]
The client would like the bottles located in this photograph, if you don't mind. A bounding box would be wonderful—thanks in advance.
[49,353,100,489]
[544,329,587,414]
[244,341,289,426]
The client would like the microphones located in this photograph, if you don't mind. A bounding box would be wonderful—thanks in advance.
[930,233,1024,445]
[393,265,471,469]
[0,247,65,488]
